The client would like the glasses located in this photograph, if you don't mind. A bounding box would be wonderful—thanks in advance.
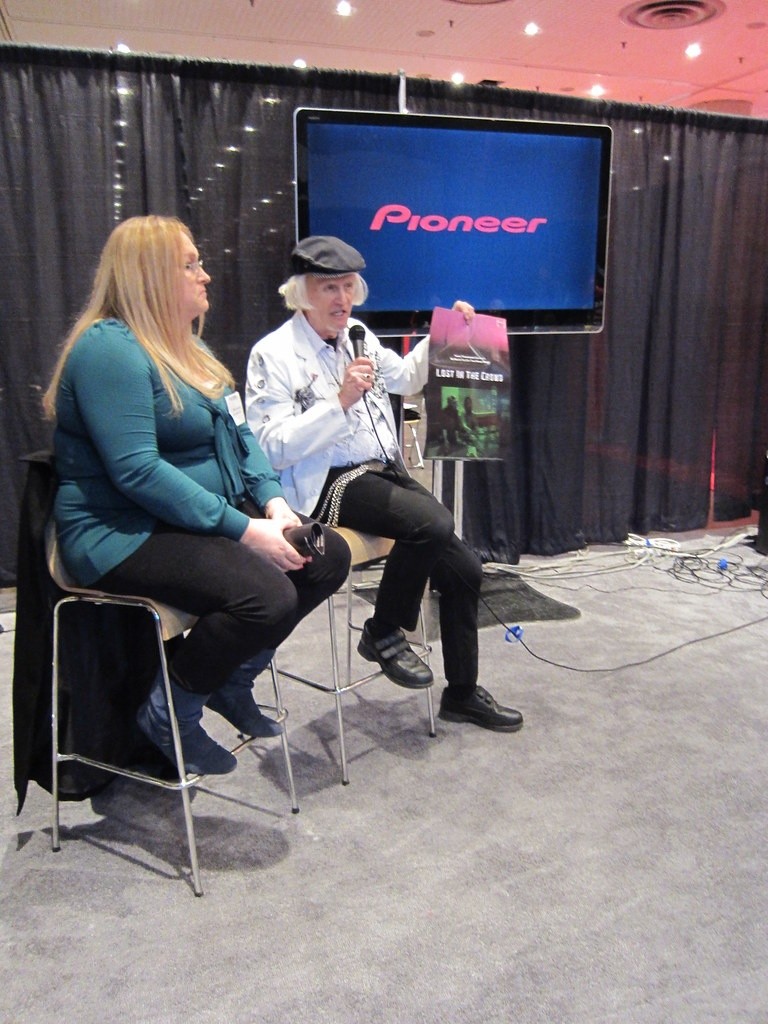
[182,259,203,271]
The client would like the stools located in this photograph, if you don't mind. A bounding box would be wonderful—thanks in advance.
[239,526,436,786]
[404,404,426,469]
[42,506,299,899]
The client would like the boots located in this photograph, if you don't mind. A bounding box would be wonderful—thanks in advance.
[204,646,283,737]
[135,662,237,774]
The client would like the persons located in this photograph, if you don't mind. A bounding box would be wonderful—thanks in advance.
[437,395,479,449]
[245,236,524,732]
[38,214,352,776]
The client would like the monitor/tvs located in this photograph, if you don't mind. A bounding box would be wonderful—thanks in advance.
[290,106,615,335]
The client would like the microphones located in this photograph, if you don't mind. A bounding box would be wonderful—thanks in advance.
[348,325,366,393]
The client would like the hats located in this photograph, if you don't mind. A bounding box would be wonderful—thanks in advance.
[290,235,367,274]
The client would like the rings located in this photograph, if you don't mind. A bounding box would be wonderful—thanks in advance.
[364,374,369,379]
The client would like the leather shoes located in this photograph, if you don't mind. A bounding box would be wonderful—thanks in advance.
[438,685,523,732]
[357,617,434,689]
